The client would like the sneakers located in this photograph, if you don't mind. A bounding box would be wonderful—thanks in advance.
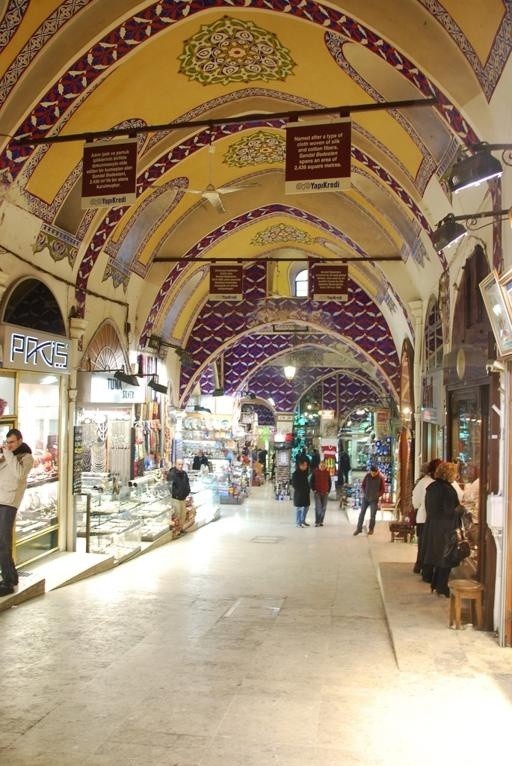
[353,527,373,536]
[0,574,18,597]
[297,522,323,528]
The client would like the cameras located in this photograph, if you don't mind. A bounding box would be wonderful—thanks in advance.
[0,448,2,454]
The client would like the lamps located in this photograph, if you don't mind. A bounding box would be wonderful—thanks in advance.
[147,334,194,369]
[84,368,138,386]
[447,142,512,192]
[431,208,508,253]
[283,331,297,382]
[130,374,168,394]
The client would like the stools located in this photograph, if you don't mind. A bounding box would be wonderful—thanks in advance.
[389,521,414,543]
[449,580,485,632]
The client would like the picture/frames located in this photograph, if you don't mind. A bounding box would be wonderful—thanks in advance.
[499,267,512,331]
[478,269,512,357]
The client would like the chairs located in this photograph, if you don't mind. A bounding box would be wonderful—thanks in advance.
[380,496,401,521]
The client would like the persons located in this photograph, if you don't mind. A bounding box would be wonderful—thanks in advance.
[418,461,462,598]
[463,467,480,524]
[407,463,430,574]
[1,428,35,588]
[291,458,311,528]
[353,464,386,537]
[309,460,332,528]
[411,459,441,583]
[337,448,351,483]
[258,450,268,466]
[163,457,192,532]
[192,450,208,471]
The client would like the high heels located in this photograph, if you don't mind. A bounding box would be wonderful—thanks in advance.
[431,583,450,598]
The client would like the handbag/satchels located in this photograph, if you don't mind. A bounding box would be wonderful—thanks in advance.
[443,527,470,562]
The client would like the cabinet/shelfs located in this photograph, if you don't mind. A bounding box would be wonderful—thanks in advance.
[0,369,68,569]
[74,411,252,564]
